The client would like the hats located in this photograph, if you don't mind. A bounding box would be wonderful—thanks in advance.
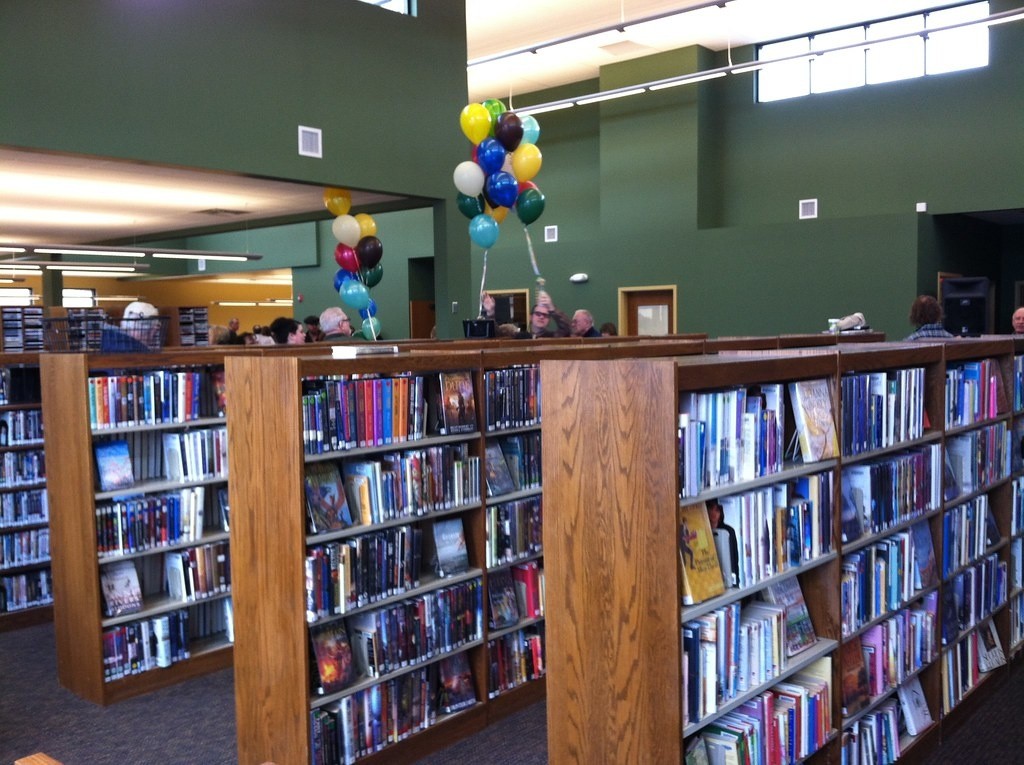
[120,301,160,329]
[304,316,319,325]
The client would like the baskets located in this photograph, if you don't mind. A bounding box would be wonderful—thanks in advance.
[40,317,168,354]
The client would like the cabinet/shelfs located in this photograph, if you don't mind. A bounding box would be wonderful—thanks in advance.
[0,305,1024,765]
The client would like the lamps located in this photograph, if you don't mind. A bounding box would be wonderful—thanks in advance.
[0,244,292,306]
[465,0,1023,118]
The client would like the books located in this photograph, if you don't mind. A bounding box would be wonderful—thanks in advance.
[300,362,546,765]
[0,362,54,612]
[0,305,210,353]
[87,365,235,683]
[676,356,1024,765]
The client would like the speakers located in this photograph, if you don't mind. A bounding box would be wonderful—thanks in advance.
[942,277,989,337]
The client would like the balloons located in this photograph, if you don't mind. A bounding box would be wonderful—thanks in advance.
[453,99,545,251]
[323,188,383,340]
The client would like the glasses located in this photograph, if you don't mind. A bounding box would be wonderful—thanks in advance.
[535,312,550,318]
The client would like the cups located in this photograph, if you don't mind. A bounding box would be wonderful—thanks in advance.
[463,319,495,339]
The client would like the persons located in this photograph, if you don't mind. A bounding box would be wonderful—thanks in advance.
[903,295,954,341]
[1013,308,1024,334]
[480,291,571,338]
[600,322,617,336]
[121,302,161,351]
[208,307,354,346]
[572,309,601,337]
